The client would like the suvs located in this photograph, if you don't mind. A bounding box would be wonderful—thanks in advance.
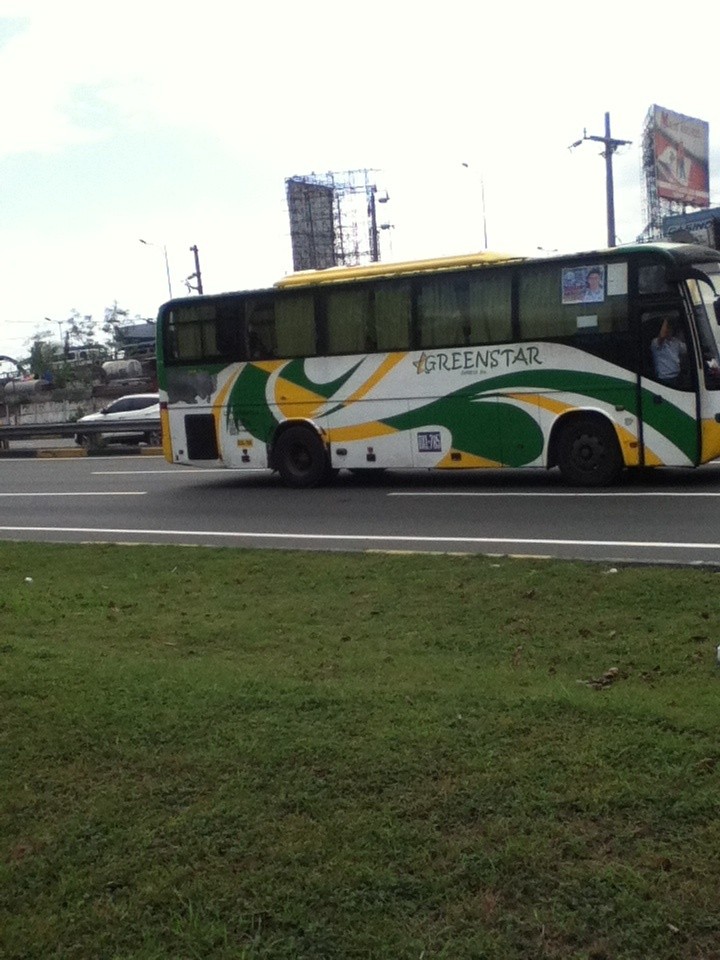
[74,391,163,448]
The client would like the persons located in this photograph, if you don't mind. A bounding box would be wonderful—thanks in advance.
[651,317,711,389]
[678,141,686,178]
[583,271,604,302]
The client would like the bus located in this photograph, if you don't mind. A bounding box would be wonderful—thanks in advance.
[154,240,720,492]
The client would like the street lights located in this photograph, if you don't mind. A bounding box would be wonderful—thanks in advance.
[138,239,172,299]
[44,317,64,347]
[462,162,489,249]
